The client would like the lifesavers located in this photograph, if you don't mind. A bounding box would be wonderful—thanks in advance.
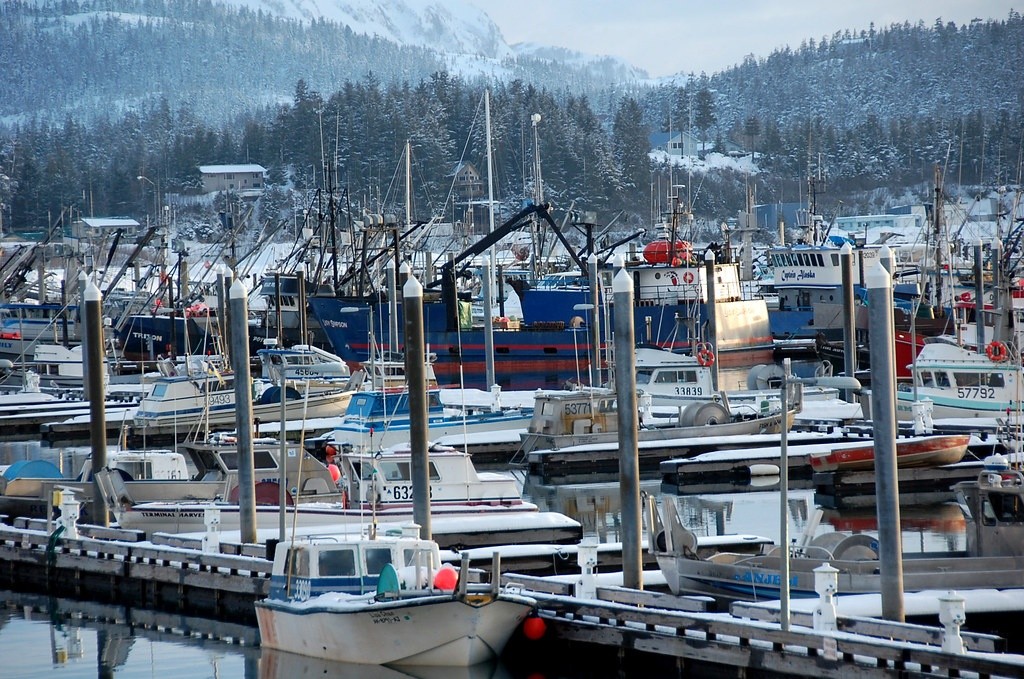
[672,257,681,266]
[697,349,716,367]
[961,292,971,301]
[683,271,695,284]
[986,340,1008,361]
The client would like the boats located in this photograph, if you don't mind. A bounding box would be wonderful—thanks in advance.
[256,381,539,668]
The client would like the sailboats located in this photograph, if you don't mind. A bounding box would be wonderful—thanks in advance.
[0,93,1023,679]
